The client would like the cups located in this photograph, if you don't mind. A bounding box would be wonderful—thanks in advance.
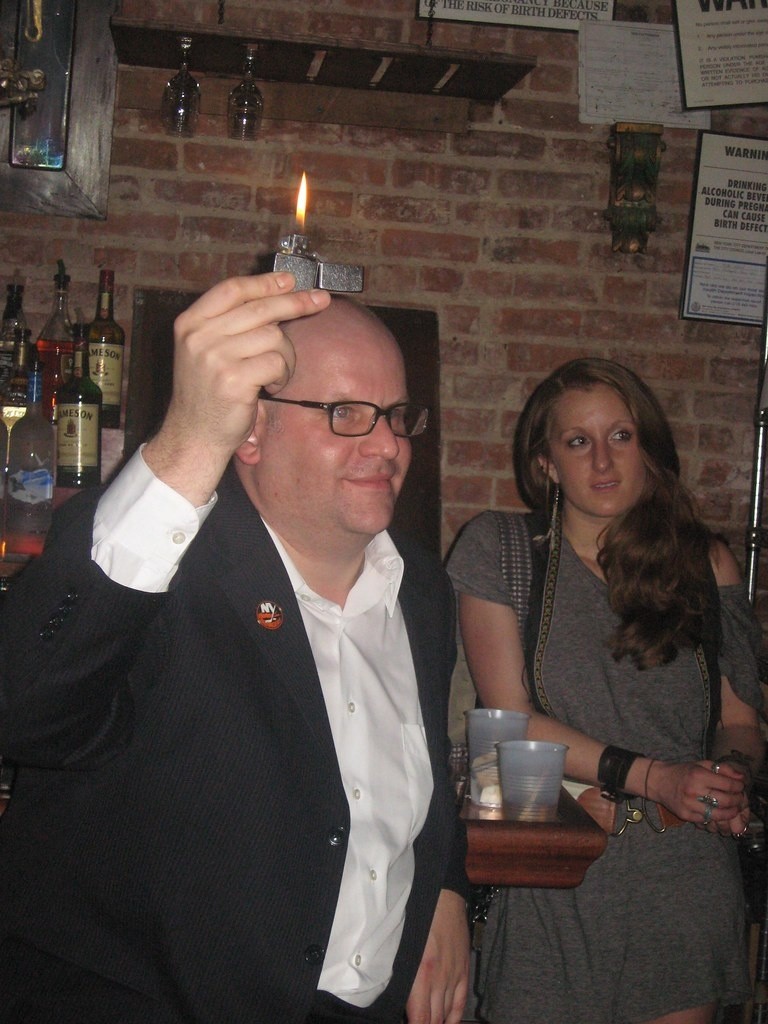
[462,706,531,808]
[495,740,570,823]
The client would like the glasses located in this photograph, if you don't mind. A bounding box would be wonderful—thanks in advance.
[259,397,430,437]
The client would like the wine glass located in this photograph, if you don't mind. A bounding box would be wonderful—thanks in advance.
[160,35,202,138]
[227,44,264,142]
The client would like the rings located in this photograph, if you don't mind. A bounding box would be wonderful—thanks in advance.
[704,806,712,824]
[733,827,747,838]
[712,762,720,773]
[697,788,718,807]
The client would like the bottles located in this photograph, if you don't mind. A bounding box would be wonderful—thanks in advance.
[0,282,28,394]
[0,328,32,473]
[35,273,74,426]
[86,269,125,428]
[0,418,8,561]
[5,361,57,563]
[56,324,103,489]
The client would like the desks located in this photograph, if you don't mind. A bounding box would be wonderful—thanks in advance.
[456,756,768,897]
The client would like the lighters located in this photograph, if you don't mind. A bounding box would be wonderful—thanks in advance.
[271,234,365,294]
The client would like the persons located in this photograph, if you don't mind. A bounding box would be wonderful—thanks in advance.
[0,273,475,1024]
[443,356,768,1024]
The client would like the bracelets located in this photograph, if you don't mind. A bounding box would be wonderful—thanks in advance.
[597,746,644,804]
[645,759,657,799]
